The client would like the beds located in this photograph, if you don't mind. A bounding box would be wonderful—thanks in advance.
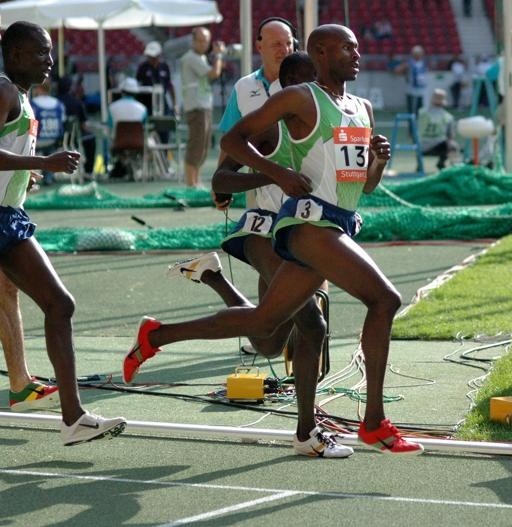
[112,122,145,180]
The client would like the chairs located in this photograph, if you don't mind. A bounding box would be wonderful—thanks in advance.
[143,119,187,180]
[61,115,102,186]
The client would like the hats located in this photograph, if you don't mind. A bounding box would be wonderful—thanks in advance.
[144,42,161,57]
[432,89,447,105]
[121,77,138,93]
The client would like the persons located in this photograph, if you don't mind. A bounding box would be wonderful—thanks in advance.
[168,52,354,457]
[106,77,147,182]
[418,87,457,171]
[174,25,226,192]
[27,58,96,185]
[122,25,426,454]
[396,45,437,135]
[452,51,505,107]
[1,22,126,446]
[136,42,177,175]
[211,17,314,354]
[0,268,64,411]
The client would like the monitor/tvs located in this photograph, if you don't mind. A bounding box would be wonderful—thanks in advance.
[107,86,154,117]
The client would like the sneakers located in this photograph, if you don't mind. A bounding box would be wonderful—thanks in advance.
[294,427,354,457]
[123,316,161,386]
[358,418,425,455]
[9,376,62,413]
[167,251,221,285]
[61,409,127,446]
